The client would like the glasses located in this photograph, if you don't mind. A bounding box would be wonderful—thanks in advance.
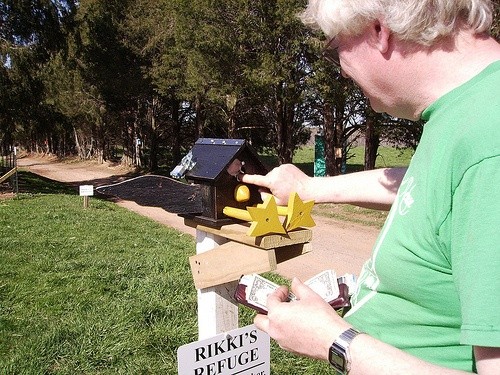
[314,29,345,67]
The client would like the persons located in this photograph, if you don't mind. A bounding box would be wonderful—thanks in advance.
[237,0,500,375]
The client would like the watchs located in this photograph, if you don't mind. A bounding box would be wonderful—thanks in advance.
[328,326,367,375]
